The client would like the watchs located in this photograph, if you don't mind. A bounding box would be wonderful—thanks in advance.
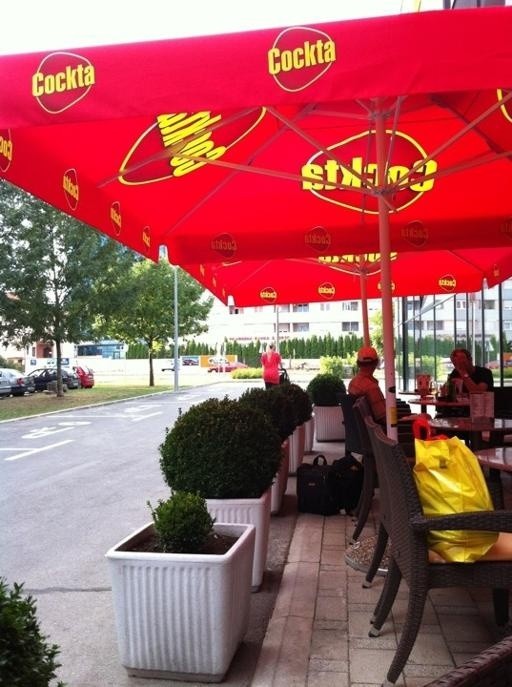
[462,374,470,379]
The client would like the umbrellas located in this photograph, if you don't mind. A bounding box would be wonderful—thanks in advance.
[1,6,512,443]
[179,248,512,347]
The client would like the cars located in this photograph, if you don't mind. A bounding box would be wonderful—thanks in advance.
[72,366,94,389]
[26,367,70,394]
[183,359,199,366]
[0,368,35,398]
[207,362,249,374]
[484,361,499,369]
[60,366,81,389]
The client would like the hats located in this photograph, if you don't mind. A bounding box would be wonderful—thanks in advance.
[357,346,378,362]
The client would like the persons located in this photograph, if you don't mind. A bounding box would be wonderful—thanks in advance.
[260,342,284,391]
[434,348,494,434]
[348,348,388,435]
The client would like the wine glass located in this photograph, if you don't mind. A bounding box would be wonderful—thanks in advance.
[429,378,464,401]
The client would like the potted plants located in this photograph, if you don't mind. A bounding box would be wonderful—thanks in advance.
[103,491,257,682]
[306,373,346,442]
[160,380,314,593]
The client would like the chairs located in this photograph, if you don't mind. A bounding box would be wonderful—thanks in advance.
[343,385,512,623]
[369,427,512,687]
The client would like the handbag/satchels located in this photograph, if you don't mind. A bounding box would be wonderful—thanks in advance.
[326,455,364,509]
[298,455,346,516]
[412,414,498,564]
